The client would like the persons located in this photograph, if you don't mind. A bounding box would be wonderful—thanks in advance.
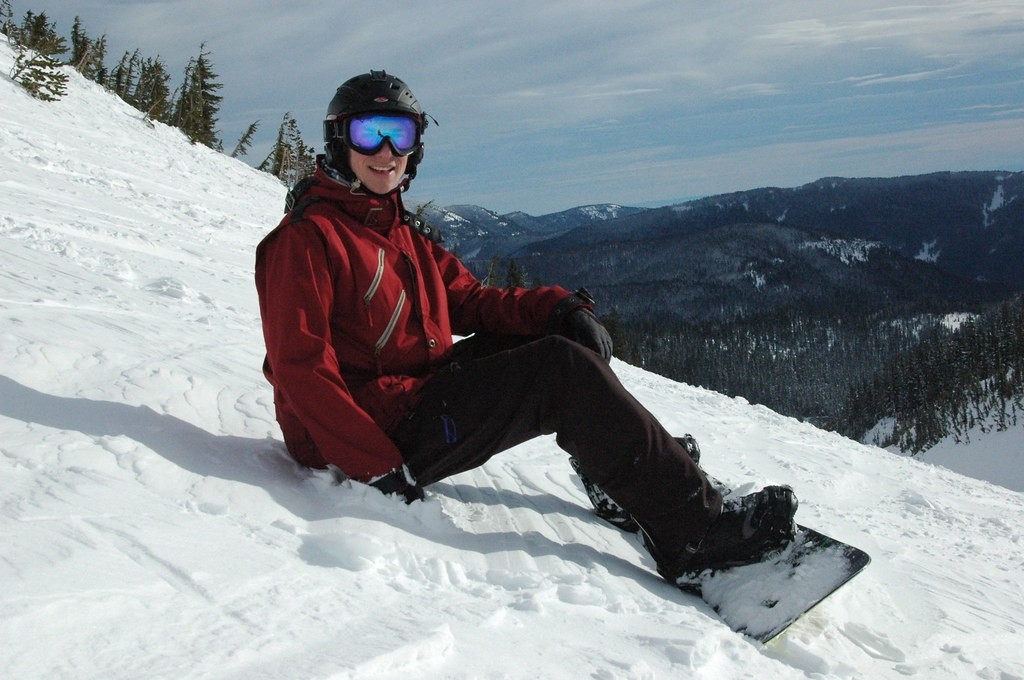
[255,69,798,590]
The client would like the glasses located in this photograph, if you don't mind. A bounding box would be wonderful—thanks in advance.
[323,113,421,157]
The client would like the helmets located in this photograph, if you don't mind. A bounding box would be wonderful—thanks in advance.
[322,68,440,199]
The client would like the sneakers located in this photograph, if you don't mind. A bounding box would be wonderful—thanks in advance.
[570,433,700,533]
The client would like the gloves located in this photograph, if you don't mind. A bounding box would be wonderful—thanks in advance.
[554,307,614,364]
[366,464,426,505]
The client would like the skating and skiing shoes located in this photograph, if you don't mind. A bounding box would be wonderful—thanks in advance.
[663,484,798,590]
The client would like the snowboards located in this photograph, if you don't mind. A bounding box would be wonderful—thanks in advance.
[633,468,872,645]
